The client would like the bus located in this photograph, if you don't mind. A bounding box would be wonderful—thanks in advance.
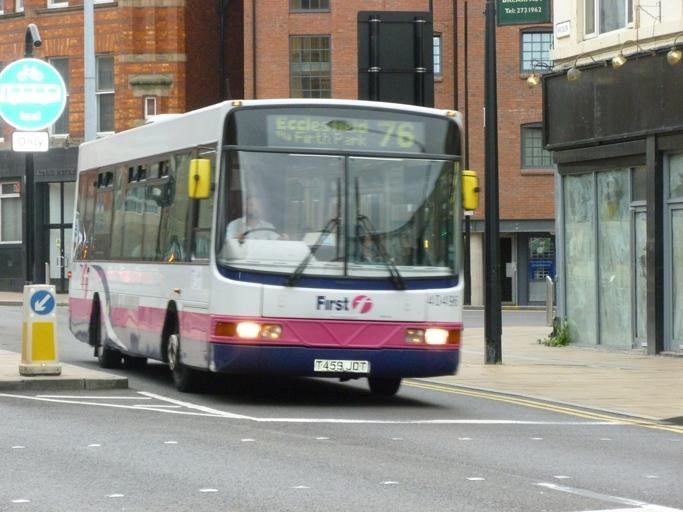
[66,97,469,401]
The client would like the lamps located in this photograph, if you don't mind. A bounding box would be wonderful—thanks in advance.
[667,32,683,66]
[526,62,553,88]
[567,54,604,80]
[612,39,653,69]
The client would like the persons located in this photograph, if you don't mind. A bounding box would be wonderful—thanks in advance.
[223,193,291,242]
